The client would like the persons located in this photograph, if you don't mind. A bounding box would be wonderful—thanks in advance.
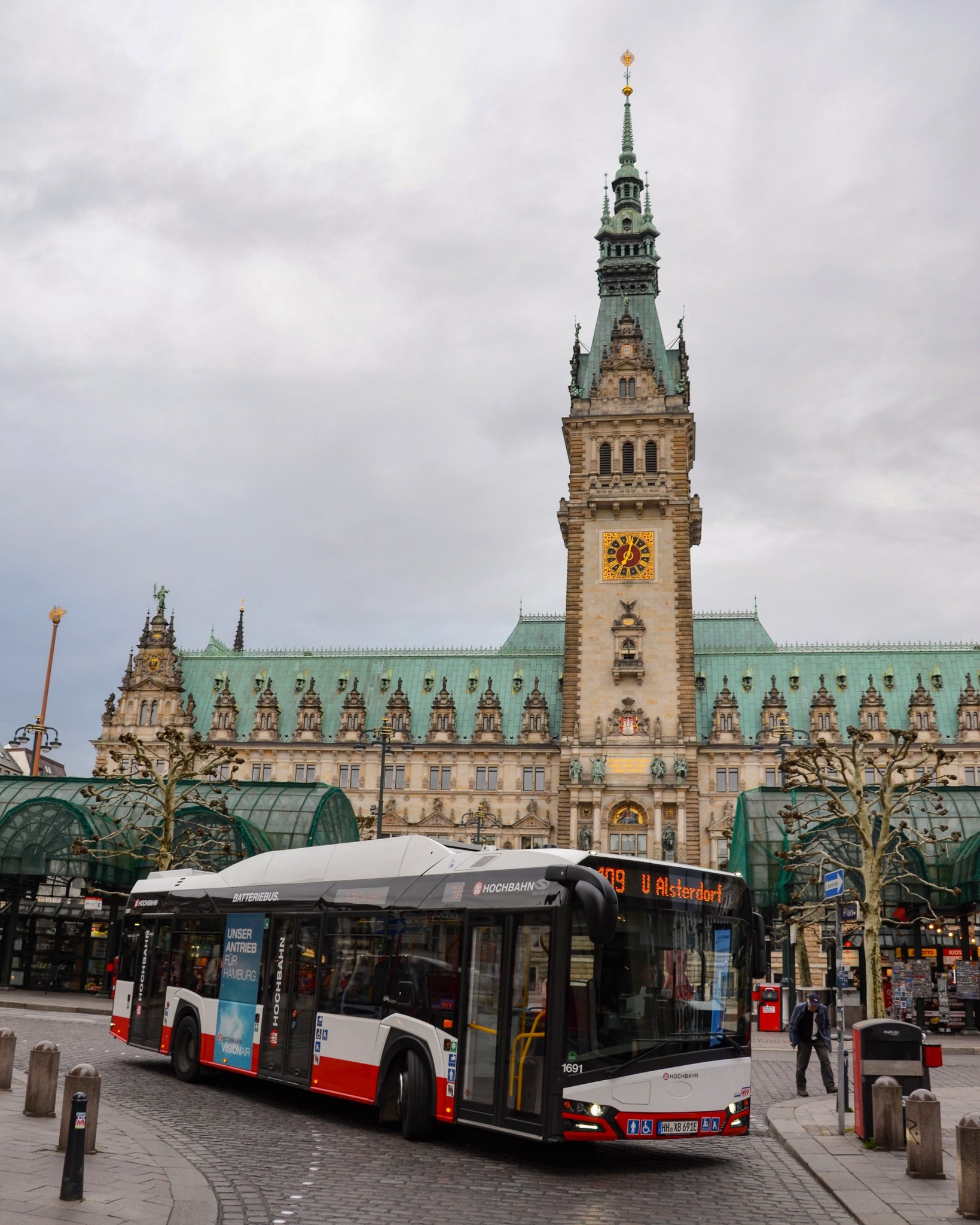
[663,823,675,853]
[588,756,606,780]
[650,755,667,779]
[789,993,837,1097]
[201,944,220,999]
[569,757,582,779]
[580,824,592,851]
[674,756,688,778]
[626,960,662,996]
[853,970,867,1021]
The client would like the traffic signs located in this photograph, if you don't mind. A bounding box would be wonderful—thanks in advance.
[822,868,846,900]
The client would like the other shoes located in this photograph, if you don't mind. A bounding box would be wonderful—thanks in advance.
[797,1089,809,1096]
[826,1086,838,1094]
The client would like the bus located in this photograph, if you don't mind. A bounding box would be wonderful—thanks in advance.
[109,834,768,1146]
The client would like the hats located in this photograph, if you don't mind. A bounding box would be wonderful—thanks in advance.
[806,994,820,1006]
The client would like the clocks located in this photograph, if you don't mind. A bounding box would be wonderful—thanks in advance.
[602,531,655,581]
